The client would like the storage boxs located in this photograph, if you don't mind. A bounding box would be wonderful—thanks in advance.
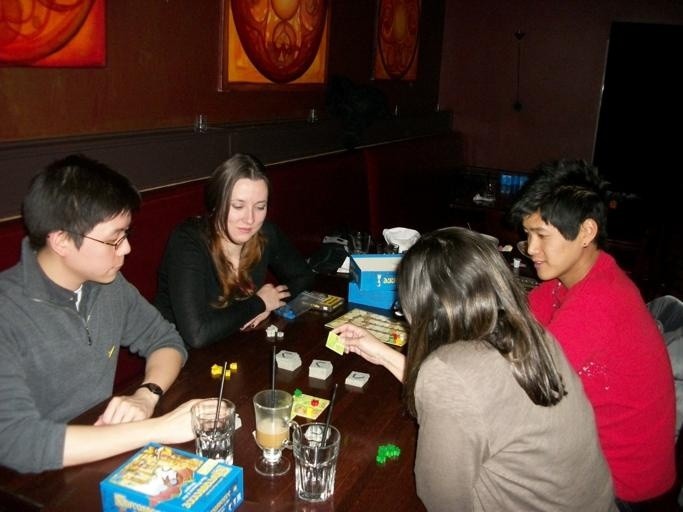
[98,442,245,512]
[350,253,405,291]
[348,280,398,310]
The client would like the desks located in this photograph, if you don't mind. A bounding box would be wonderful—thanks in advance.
[0,275,423,512]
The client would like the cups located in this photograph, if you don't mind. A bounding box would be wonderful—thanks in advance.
[191,110,208,135]
[189,398,235,467]
[348,231,370,255]
[292,422,340,504]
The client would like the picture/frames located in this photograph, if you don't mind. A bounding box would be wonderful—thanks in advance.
[0,0,108,67]
[369,0,424,87]
[218,0,330,98]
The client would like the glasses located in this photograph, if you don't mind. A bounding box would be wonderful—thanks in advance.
[62,227,133,251]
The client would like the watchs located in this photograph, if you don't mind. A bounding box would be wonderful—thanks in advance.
[142,381,165,400]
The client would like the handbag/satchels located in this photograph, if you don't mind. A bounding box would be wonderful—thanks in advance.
[304,231,358,276]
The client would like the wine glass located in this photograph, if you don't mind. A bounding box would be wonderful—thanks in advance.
[252,389,302,477]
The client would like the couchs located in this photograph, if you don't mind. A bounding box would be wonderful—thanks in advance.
[0,134,464,484]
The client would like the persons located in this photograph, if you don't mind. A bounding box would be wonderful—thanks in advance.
[152,151,315,358]
[327,226,620,512]
[0,152,243,475]
[508,156,683,512]
